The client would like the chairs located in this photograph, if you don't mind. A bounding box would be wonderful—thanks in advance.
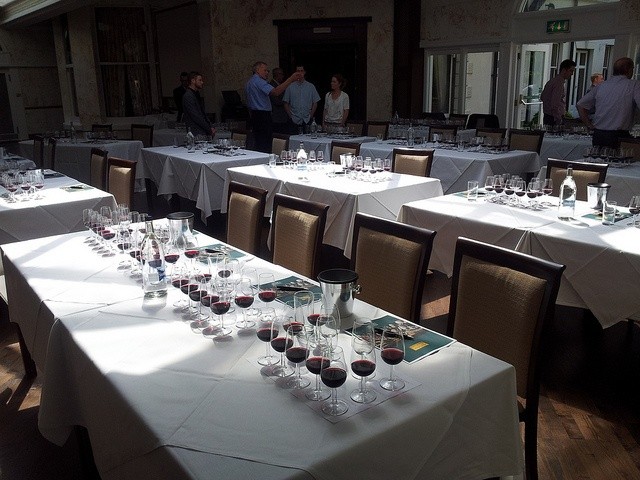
[467,114,499,129]
[232,130,248,148]
[620,138,640,161]
[33,135,44,168]
[329,141,361,164]
[92,124,112,139]
[390,147,434,177]
[131,125,154,147]
[89,148,108,192]
[360,138,543,195]
[270,133,289,155]
[269,193,330,284]
[347,212,437,325]
[346,120,366,138]
[450,114,468,127]
[448,237,566,480]
[47,138,56,171]
[223,179,267,258]
[208,112,217,122]
[422,112,445,121]
[222,114,248,132]
[475,128,507,149]
[544,158,610,201]
[428,126,458,144]
[107,157,137,223]
[508,128,546,184]
[368,123,387,140]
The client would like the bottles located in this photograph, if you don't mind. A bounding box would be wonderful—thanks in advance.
[187,127,195,153]
[297,142,308,180]
[312,116,317,139]
[142,214,168,295]
[280,148,310,170]
[558,168,577,220]
[407,123,414,147]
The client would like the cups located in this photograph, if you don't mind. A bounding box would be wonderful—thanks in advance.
[292,290,315,333]
[602,200,618,225]
[269,153,277,169]
[468,180,478,201]
[202,145,208,155]
[377,134,383,144]
[457,140,463,152]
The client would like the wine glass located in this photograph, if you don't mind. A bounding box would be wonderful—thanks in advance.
[584,145,635,168]
[0,160,44,199]
[531,178,546,208]
[210,291,232,338]
[255,308,280,366]
[341,156,351,180]
[256,274,277,322]
[349,156,384,184]
[317,151,324,170]
[194,257,215,284]
[118,227,130,260]
[211,138,247,157]
[526,182,538,208]
[181,266,198,313]
[318,124,357,139]
[83,203,117,258]
[130,236,141,276]
[309,150,316,170]
[321,303,343,354]
[138,212,149,237]
[627,195,639,232]
[200,280,219,327]
[319,343,348,414]
[468,136,510,154]
[285,320,311,388]
[187,275,210,321]
[379,325,405,390]
[233,278,254,328]
[173,263,188,306]
[542,179,553,207]
[313,316,337,358]
[384,159,392,182]
[351,318,376,379]
[271,315,295,376]
[484,173,526,207]
[304,329,332,401]
[346,344,377,403]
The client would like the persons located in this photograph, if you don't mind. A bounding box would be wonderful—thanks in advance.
[586,73,604,95]
[541,59,576,127]
[322,75,350,133]
[244,61,301,153]
[269,68,283,133]
[182,74,216,139]
[172,71,190,126]
[575,58,640,149]
[282,66,321,133]
